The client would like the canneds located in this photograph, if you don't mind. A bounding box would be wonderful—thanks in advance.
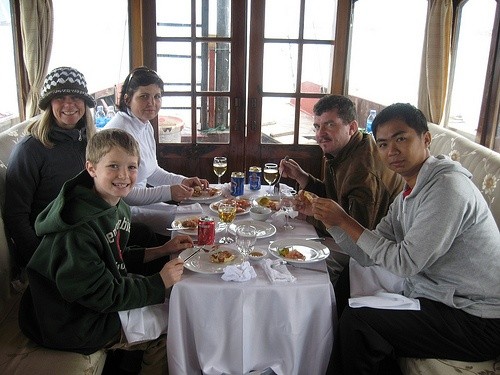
[231,172,244,195]
[249,166,261,191]
[198,217,215,246]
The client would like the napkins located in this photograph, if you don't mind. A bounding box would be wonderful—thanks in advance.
[348,256,421,310]
[177,202,203,214]
[261,259,294,282]
[118,300,169,343]
[221,262,256,282]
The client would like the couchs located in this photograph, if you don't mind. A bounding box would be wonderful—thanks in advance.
[343,120,500,375]
[0,113,107,375]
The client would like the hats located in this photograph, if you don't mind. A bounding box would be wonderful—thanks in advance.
[37,67,96,110]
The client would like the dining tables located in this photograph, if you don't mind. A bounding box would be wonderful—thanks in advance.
[166,182,337,375]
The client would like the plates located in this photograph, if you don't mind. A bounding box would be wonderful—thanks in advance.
[209,199,252,216]
[171,215,226,236]
[232,219,276,238]
[268,237,331,265]
[249,248,267,261]
[178,244,244,274]
[184,186,222,200]
[253,196,293,212]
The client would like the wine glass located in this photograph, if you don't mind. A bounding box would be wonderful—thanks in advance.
[218,200,236,244]
[212,156,228,189]
[264,162,279,194]
[237,225,256,263]
[280,196,296,230]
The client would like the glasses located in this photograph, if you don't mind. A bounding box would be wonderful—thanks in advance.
[126,67,159,95]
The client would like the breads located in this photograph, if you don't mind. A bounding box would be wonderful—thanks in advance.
[192,186,202,197]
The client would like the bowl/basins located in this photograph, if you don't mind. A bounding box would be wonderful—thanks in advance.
[249,206,272,222]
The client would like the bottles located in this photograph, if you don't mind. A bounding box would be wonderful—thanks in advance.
[105,106,116,125]
[366,110,376,134]
[94,106,106,132]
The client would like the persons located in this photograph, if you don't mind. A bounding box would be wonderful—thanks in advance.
[293,102,500,375]
[17,128,194,375]
[279,94,407,290]
[103,67,209,238]
[0,67,163,287]
